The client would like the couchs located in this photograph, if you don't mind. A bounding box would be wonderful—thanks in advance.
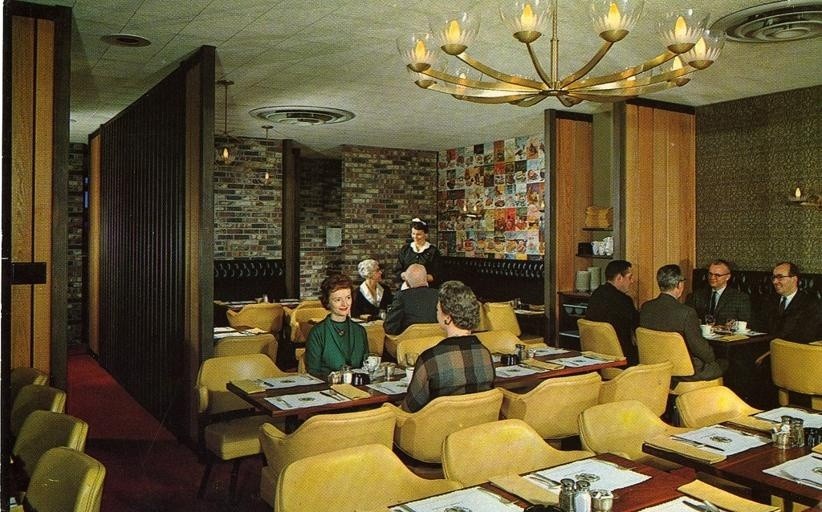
[692,269,822,330]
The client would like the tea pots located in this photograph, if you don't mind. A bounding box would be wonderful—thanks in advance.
[591,235,614,256]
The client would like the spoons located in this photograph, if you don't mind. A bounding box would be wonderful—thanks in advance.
[329,391,345,401]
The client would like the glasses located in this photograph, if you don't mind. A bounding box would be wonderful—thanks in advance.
[677,278,686,288]
[770,275,793,281]
[708,272,729,277]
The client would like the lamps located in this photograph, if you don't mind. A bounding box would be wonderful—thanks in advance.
[396,1,727,108]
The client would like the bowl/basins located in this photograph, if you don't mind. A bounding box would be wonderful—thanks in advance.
[575,267,603,293]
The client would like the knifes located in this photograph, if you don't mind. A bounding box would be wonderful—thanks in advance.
[682,500,708,512]
[322,390,341,401]
[671,435,725,452]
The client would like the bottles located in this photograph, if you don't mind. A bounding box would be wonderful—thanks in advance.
[559,478,577,512]
[780,415,805,448]
[341,365,353,384]
[574,480,591,512]
[514,344,527,360]
[378,309,386,320]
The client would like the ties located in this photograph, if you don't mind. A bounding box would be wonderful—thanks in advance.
[780,297,787,316]
[711,291,716,314]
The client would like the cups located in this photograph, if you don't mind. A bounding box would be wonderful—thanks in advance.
[700,325,714,335]
[733,321,748,332]
[406,367,415,382]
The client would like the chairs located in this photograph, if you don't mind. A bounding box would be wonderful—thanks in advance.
[676,386,764,427]
[25,448,106,512]
[771,337,822,411]
[274,444,462,512]
[441,418,595,487]
[599,360,674,418]
[194,353,299,512]
[577,318,627,379]
[259,404,397,512]
[577,400,700,464]
[383,387,504,479]
[11,367,89,481]
[636,327,724,398]
[498,372,601,448]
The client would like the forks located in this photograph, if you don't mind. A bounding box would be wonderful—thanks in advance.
[276,396,292,408]
[780,469,822,488]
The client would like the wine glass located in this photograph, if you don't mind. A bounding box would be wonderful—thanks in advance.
[405,352,420,367]
[725,319,739,336]
[363,353,380,381]
[705,315,716,327]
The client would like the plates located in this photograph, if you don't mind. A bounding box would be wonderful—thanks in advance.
[400,376,411,384]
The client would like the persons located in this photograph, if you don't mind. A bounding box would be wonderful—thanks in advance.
[383,263,439,336]
[394,216,442,288]
[305,273,369,374]
[585,260,639,365]
[685,257,754,324]
[351,257,392,317]
[766,260,821,346]
[401,279,496,415]
[640,263,746,390]
